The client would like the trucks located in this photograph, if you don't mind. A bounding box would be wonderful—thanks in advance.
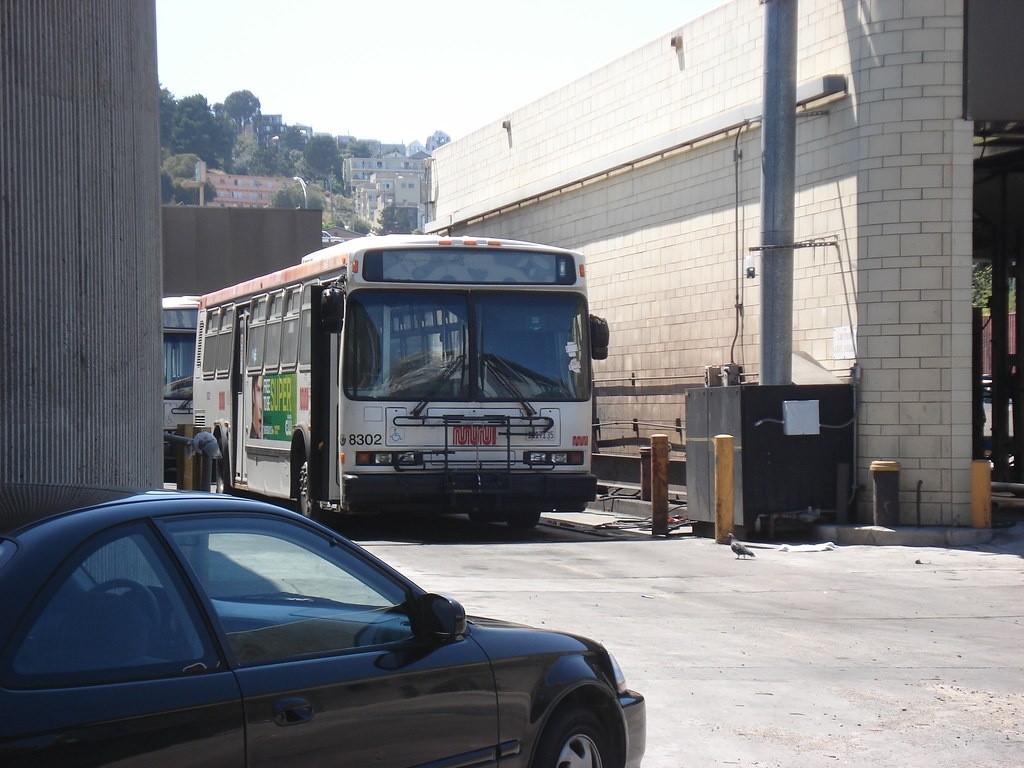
[982,313,1017,403]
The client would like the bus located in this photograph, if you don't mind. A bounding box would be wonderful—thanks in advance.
[194,232,611,528]
[162,296,217,475]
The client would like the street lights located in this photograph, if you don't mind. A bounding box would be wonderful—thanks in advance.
[194,160,207,205]
[293,175,309,209]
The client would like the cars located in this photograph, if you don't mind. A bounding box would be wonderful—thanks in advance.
[322,230,345,243]
[1,493,645,768]
